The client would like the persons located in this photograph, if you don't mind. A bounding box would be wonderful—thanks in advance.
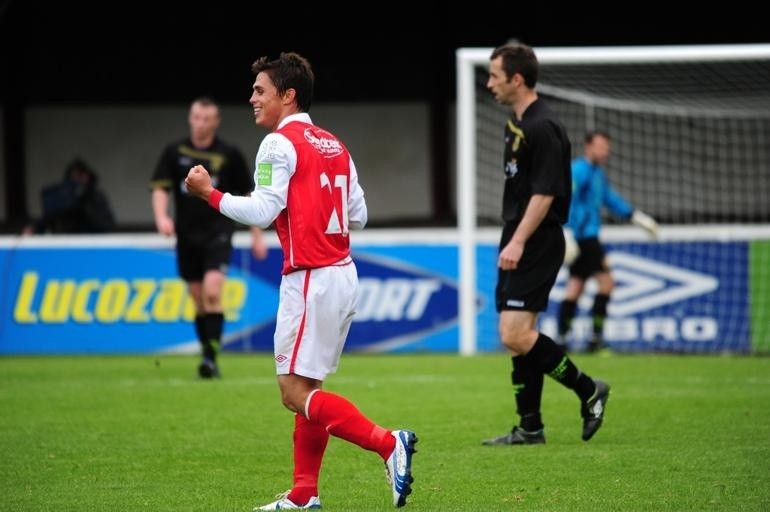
[143,97,267,377]
[183,53,418,512]
[554,130,660,353]
[481,38,610,445]
[20,157,121,235]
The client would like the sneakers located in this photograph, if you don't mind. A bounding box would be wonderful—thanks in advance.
[253,495,324,512]
[580,380,611,441]
[481,426,547,447]
[198,361,221,379]
[584,340,613,360]
[384,429,418,509]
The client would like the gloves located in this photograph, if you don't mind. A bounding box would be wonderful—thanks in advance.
[629,208,662,241]
[562,226,582,268]
